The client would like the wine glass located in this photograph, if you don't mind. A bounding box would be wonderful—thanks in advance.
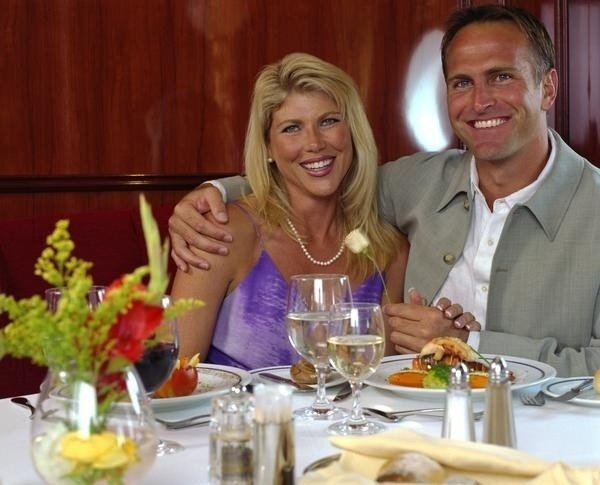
[326,303,387,438]
[285,274,350,420]
[134,297,185,458]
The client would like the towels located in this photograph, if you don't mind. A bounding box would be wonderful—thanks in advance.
[292,426,600,485]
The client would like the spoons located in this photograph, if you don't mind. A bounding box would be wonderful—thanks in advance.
[365,407,483,425]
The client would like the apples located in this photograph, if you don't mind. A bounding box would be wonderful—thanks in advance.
[155,356,198,397]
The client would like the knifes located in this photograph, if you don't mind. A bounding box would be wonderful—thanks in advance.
[260,372,317,392]
[551,380,595,401]
[330,382,369,402]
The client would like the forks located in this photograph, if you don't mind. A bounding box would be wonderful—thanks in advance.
[519,390,548,407]
[10,397,58,422]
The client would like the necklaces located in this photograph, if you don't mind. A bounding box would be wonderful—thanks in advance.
[286,217,347,267]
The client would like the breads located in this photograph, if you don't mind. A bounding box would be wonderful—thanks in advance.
[289,359,330,384]
[377,452,482,484]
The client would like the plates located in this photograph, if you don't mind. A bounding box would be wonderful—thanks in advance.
[304,452,342,473]
[247,360,346,393]
[361,352,555,400]
[540,375,599,408]
[38,362,251,413]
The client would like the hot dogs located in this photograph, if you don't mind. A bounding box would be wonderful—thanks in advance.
[386,335,515,388]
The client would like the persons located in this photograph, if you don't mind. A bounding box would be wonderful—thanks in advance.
[156,51,483,374]
[166,4,600,379]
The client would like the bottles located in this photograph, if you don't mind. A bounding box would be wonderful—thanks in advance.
[206,385,294,485]
[486,357,519,449]
[441,361,476,441]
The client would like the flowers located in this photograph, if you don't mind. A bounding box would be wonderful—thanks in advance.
[0,192,206,485]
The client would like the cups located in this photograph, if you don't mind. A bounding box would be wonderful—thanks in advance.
[27,361,161,485]
[45,286,106,324]
